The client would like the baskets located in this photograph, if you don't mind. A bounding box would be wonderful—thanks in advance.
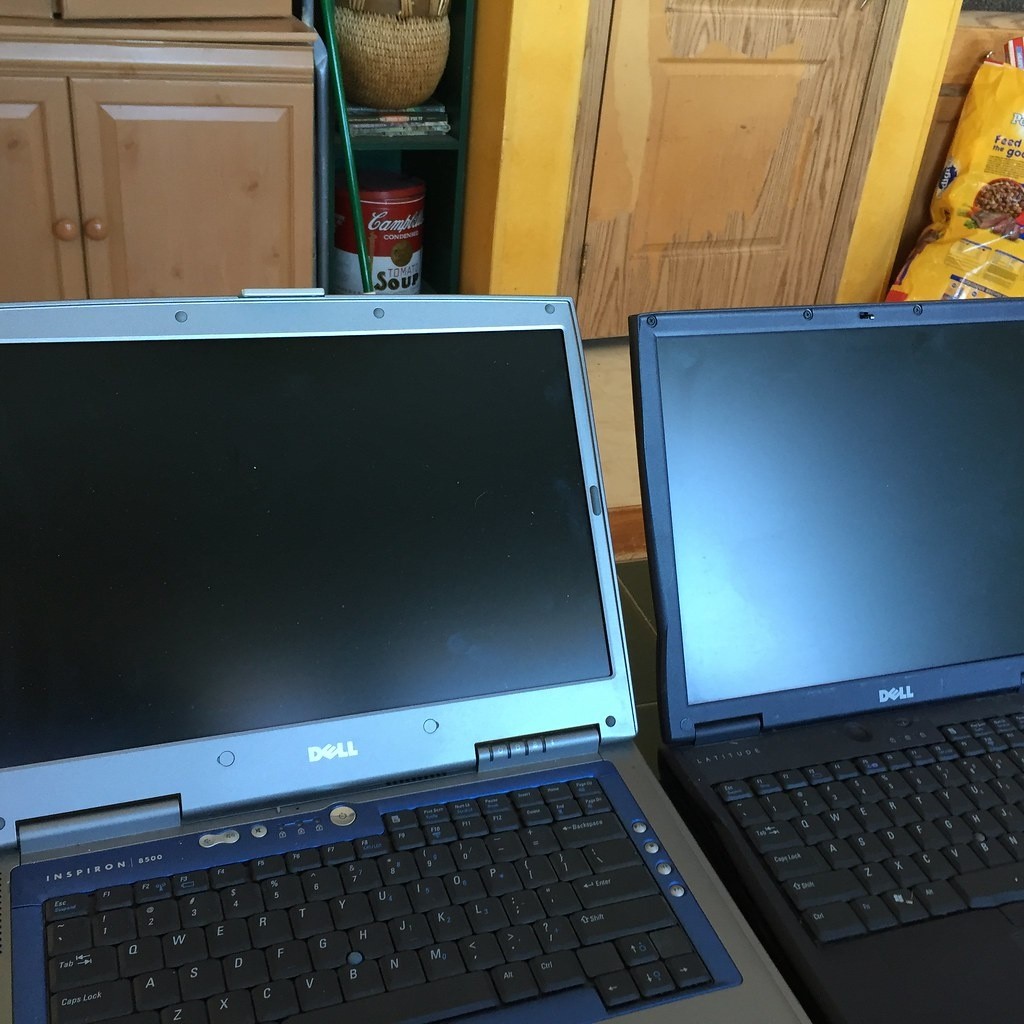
[336,0,450,109]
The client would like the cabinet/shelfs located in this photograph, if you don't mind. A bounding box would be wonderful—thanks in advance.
[0,16,318,304]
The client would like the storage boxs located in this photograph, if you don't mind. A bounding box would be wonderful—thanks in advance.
[57,0,292,21]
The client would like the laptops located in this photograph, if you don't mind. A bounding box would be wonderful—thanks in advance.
[629,294,1023,1023]
[1,287,812,1024]
